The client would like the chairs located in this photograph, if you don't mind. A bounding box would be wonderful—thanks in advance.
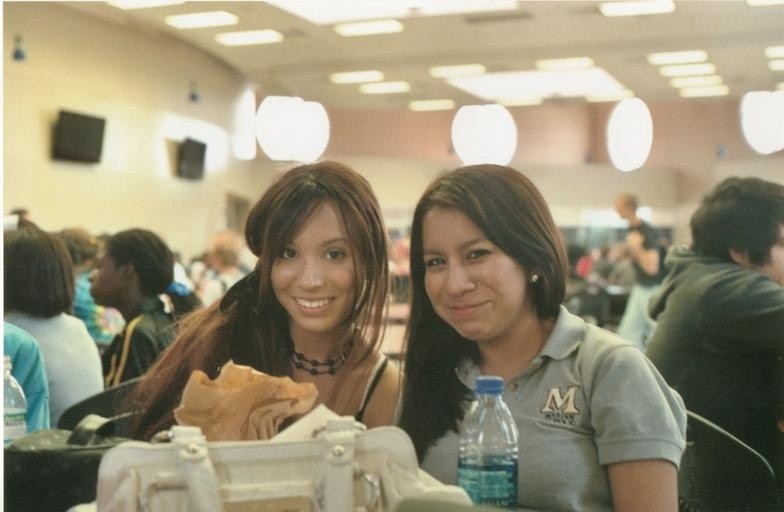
[57,373,151,440]
[679,410,776,512]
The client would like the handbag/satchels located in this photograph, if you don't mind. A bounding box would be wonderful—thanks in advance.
[5,402,129,512]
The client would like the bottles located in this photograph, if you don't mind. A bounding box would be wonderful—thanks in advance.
[455,375,520,511]
[3,355,27,453]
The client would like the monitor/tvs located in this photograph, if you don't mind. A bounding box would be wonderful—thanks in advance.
[177,138,207,178]
[52,109,106,163]
